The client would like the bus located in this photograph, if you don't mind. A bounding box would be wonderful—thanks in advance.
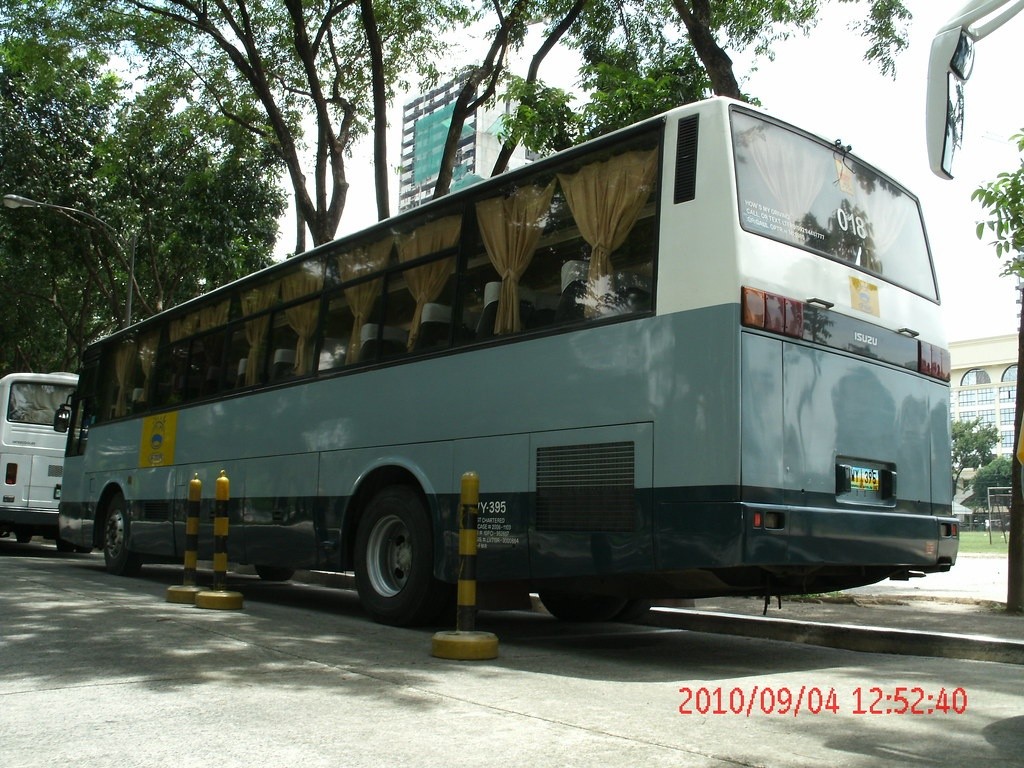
[53,100,961,626]
[0,370,80,544]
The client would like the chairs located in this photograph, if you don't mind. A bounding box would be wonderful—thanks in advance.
[130,260,592,415]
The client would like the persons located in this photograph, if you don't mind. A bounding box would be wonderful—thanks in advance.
[984,517,989,536]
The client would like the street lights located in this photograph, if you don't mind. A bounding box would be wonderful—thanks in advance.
[4,193,137,328]
